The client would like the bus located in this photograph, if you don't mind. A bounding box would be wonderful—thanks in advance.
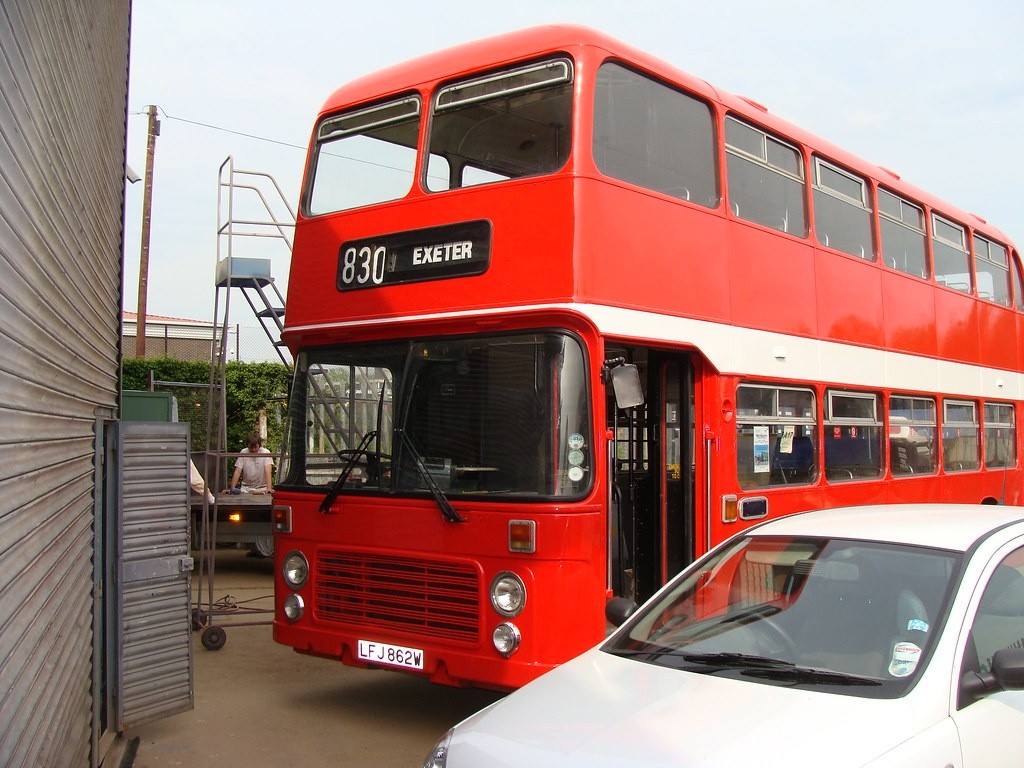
[271,25,1024,695]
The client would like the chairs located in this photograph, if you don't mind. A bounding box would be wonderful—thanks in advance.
[964,564,1024,671]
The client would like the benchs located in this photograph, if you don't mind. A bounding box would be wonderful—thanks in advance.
[771,434,1014,484]
[650,189,1023,312]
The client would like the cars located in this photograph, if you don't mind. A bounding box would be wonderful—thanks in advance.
[427,501,1024,768]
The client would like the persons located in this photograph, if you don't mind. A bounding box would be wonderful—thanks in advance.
[230,433,274,494]
[190,457,215,505]
